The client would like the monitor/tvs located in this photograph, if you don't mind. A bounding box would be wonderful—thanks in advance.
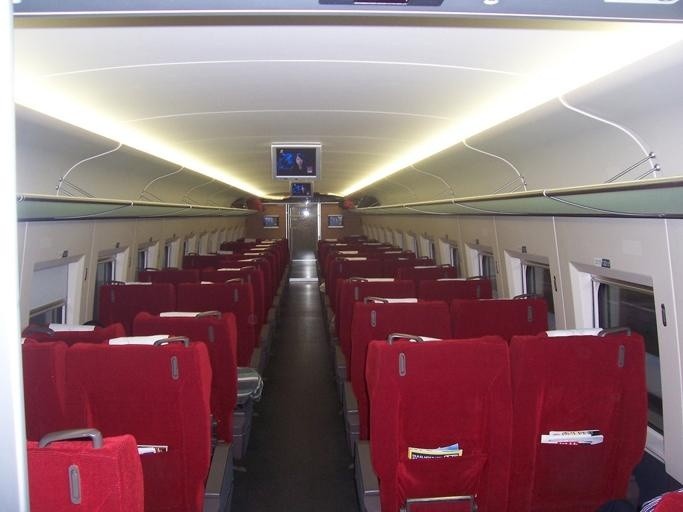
[271,142,320,181]
[264,215,280,228]
[289,181,314,198]
[328,215,344,229]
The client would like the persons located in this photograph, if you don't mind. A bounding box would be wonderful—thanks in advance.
[291,152,308,172]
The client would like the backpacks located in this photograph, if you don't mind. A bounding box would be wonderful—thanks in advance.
[343,196,381,208]
[247,196,263,211]
[232,198,249,209]
